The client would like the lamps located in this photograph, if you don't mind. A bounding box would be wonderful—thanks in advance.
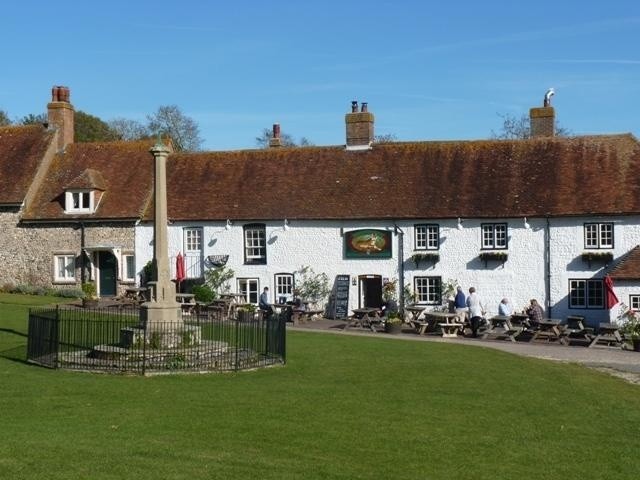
[282,218,291,231]
[223,218,232,230]
[454,218,464,230]
[393,221,406,236]
[521,216,533,229]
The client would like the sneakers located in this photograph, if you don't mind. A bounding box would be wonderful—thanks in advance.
[459,331,464,335]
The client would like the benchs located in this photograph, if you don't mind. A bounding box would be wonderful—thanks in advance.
[341,304,640,350]
[110,288,326,328]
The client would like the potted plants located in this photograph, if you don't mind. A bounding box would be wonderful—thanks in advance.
[79,284,100,310]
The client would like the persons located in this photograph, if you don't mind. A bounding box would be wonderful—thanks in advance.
[499,298,510,331]
[466,287,485,323]
[527,299,543,329]
[259,286,273,318]
[454,286,466,335]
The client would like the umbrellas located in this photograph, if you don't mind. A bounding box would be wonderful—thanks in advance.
[176,251,186,293]
[604,273,618,324]
